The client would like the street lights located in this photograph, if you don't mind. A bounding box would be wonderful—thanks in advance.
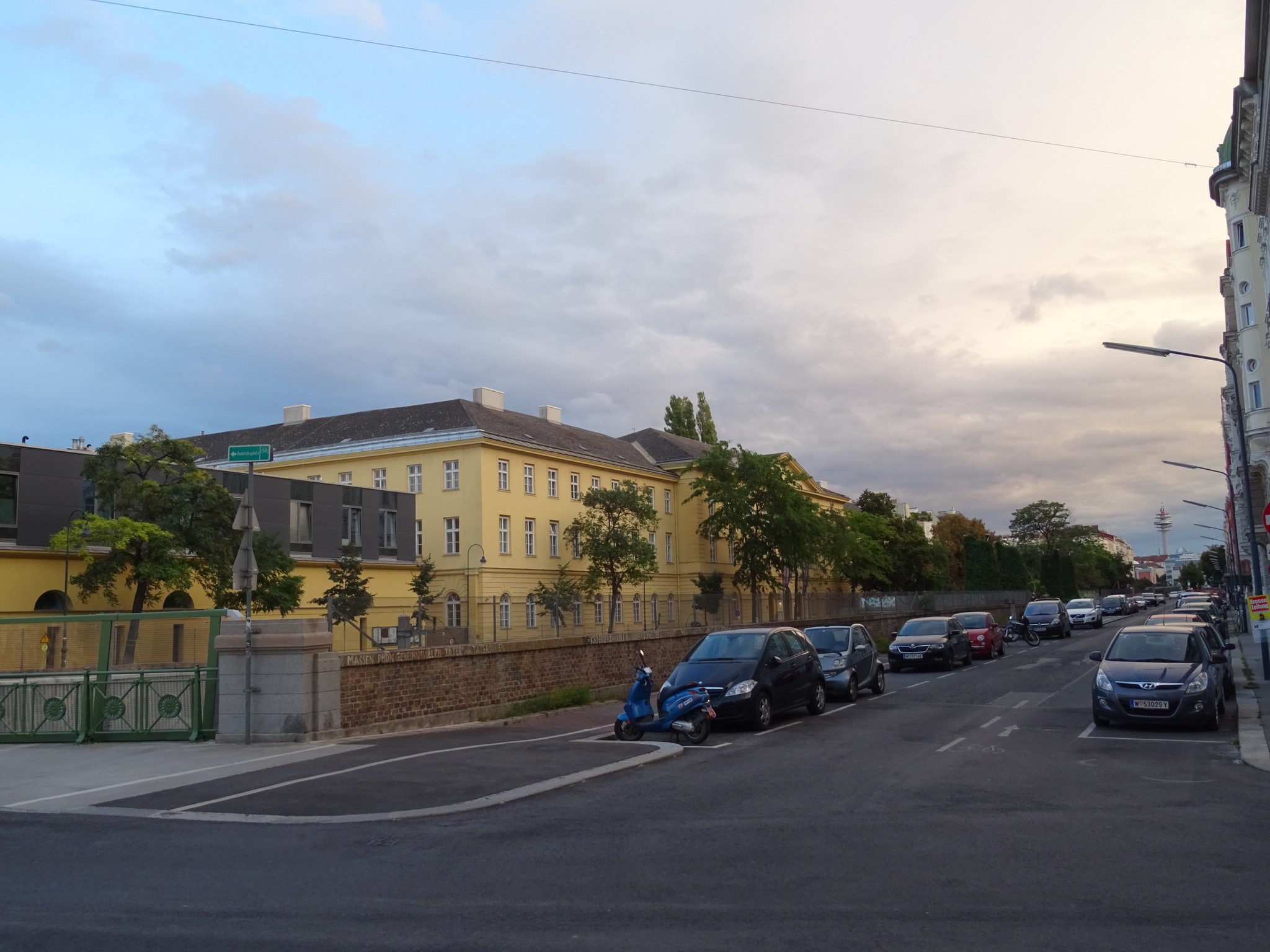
[60,509,91,667]
[466,544,486,643]
[1198,535,1237,609]
[1193,523,1237,610]
[1103,342,1270,682]
[1182,500,1239,612]
[1161,460,1248,632]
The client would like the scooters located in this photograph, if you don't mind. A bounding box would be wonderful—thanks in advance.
[614,649,717,743]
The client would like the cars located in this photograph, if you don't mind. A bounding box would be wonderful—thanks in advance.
[1140,593,1166,606]
[1089,614,1235,731]
[1066,597,1102,629]
[1022,598,1071,639]
[1098,595,1130,616]
[1169,592,1229,636]
[951,611,1005,658]
[657,627,826,731]
[1168,591,1179,599]
[1131,596,1147,610]
[792,624,885,701]
[888,616,973,671]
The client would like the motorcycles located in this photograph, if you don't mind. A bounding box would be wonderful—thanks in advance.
[996,616,1041,647]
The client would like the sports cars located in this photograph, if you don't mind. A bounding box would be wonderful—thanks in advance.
[1127,597,1138,613]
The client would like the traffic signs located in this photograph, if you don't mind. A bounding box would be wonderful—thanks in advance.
[227,444,272,462]
